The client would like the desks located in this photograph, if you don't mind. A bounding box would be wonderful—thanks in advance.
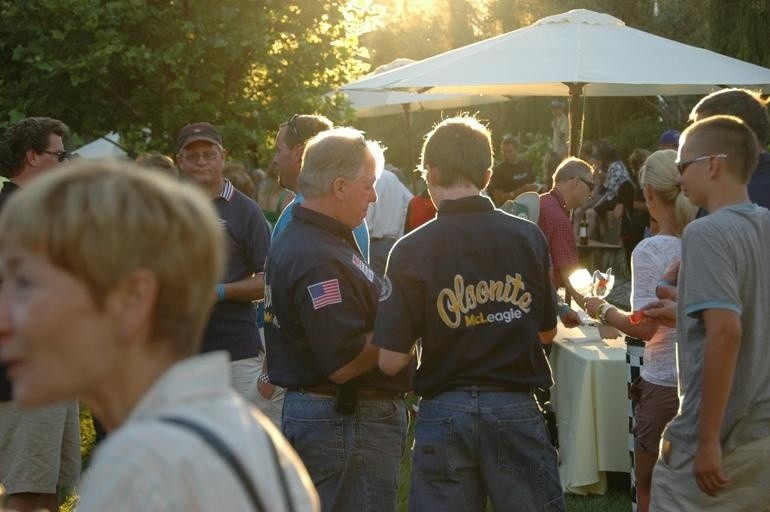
[538,313,640,500]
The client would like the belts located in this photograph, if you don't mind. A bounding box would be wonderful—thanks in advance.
[302,381,410,400]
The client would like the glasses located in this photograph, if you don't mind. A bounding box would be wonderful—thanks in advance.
[288,114,301,143]
[45,150,67,162]
[181,151,218,162]
[571,130,729,190]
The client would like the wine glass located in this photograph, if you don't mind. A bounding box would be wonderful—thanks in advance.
[569,268,602,326]
[588,274,615,322]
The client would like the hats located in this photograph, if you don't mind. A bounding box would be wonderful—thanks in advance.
[177,122,222,149]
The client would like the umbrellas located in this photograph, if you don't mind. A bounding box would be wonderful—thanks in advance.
[340,4,770,158]
[318,58,510,199]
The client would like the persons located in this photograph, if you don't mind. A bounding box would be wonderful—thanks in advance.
[140,87,770,512]
[0,113,85,511]
[0,155,323,512]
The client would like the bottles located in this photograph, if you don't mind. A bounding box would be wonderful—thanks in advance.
[579,213,588,245]
